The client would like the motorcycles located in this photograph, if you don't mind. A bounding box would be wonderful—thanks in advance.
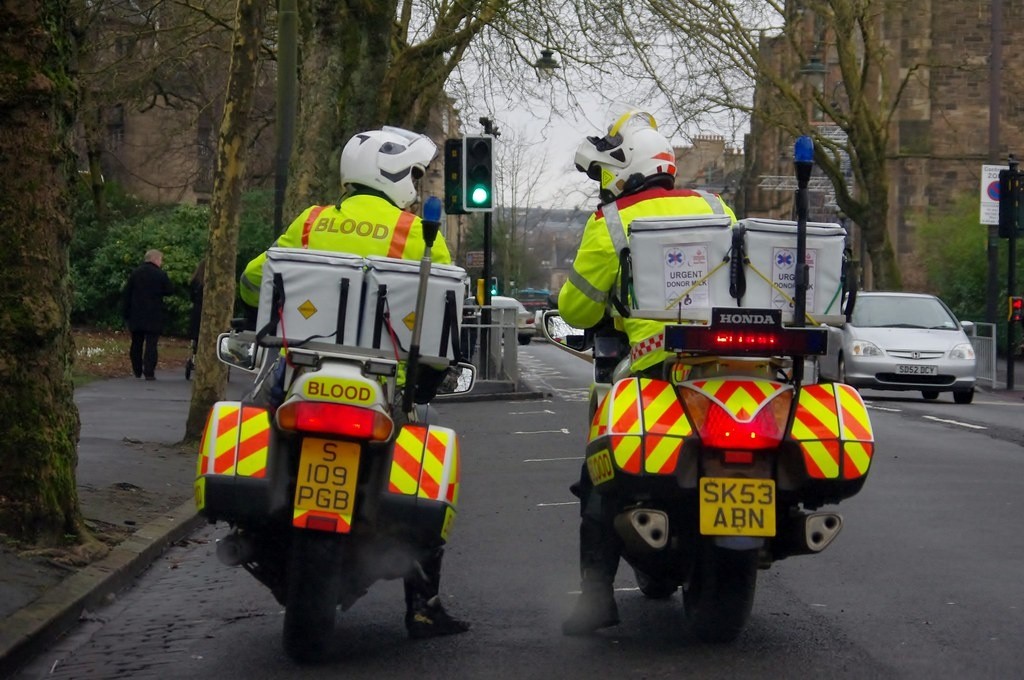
[541,135,875,646]
[194,195,477,662]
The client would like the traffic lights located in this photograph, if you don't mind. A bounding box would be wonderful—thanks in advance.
[443,136,492,215]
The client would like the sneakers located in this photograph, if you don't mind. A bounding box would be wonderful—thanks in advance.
[562,578,621,636]
[404,598,471,640]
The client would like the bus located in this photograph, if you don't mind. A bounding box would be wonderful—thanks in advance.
[517,287,553,314]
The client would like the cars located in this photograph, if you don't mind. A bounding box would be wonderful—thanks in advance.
[812,291,979,404]
[464,296,537,345]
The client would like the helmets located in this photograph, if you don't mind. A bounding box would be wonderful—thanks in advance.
[340,126,440,209]
[574,110,677,204]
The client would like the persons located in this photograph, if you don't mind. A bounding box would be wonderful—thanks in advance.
[240,124,471,640]
[125,247,171,382]
[556,110,742,639]
[183,257,208,381]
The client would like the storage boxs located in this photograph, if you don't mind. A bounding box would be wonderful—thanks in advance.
[733,218,847,318]
[358,255,466,362]
[254,247,365,348]
[627,214,739,316]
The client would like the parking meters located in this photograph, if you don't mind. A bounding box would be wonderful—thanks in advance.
[1007,296,1024,322]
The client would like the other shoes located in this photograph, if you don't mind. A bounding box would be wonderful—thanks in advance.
[146,377,157,381]
[185,358,195,380]
